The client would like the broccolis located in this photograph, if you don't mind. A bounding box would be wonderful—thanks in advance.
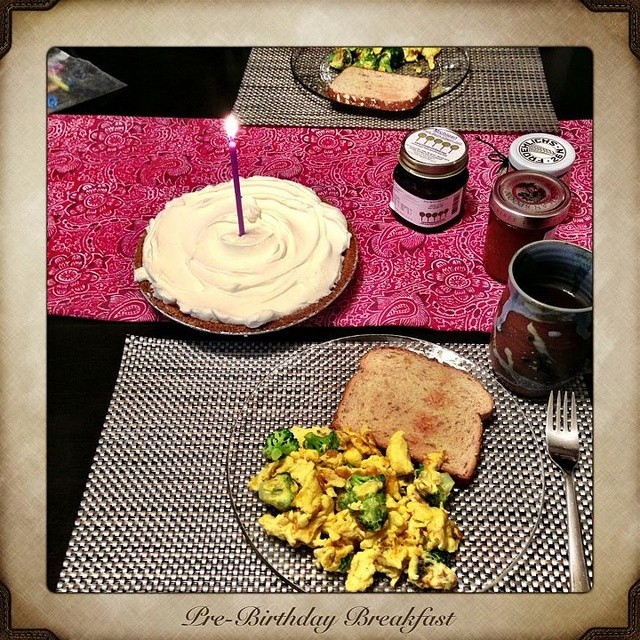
[328,46,440,73]
[252,427,461,593]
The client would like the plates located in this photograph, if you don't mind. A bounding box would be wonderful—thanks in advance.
[286,43,483,116]
[225,330,549,593]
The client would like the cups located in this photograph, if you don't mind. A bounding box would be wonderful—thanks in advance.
[491,238,596,403]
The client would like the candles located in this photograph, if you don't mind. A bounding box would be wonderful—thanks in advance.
[228,134,246,236]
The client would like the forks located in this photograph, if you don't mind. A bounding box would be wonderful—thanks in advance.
[544,389,593,593]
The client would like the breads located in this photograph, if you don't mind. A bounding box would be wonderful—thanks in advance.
[327,66,432,112]
[328,345,495,482]
